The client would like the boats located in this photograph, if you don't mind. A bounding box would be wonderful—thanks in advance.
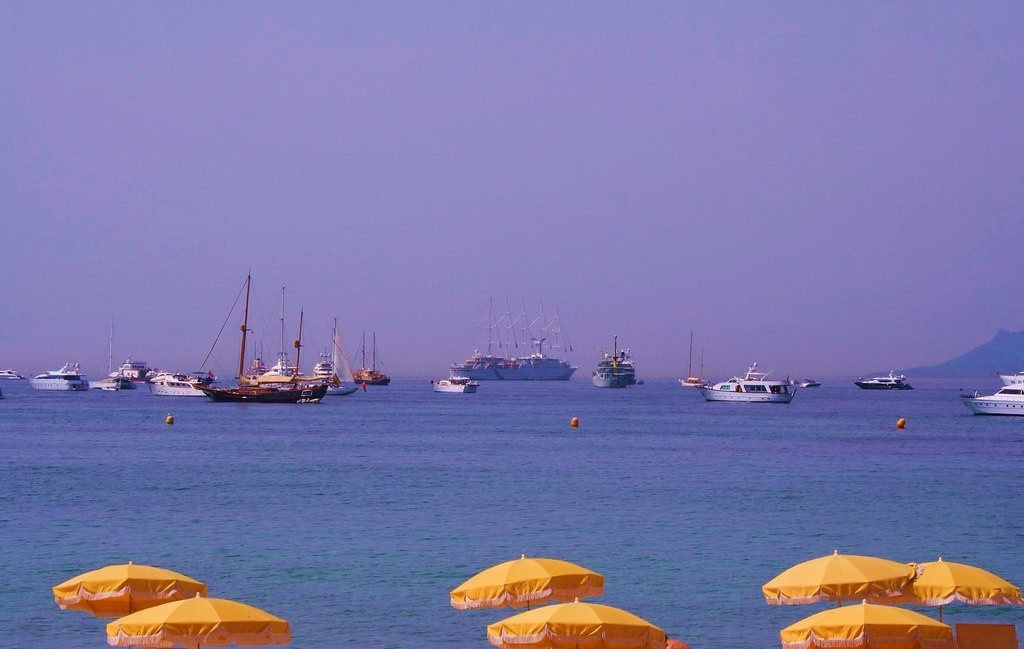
[799,378,822,388]
[958,383,1024,416]
[694,361,800,404]
[31,360,90,392]
[0,366,25,381]
[592,335,635,389]
[785,372,798,386]
[430,370,481,394]
[998,370,1024,386]
[88,314,224,399]
[854,369,914,390]
[296,394,321,404]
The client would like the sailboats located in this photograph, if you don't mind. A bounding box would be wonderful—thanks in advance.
[678,330,708,388]
[189,266,337,404]
[245,286,359,397]
[349,328,391,386]
[449,295,582,381]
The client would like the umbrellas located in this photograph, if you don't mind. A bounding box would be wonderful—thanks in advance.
[761,550,923,606]
[487,594,666,649]
[50,559,208,615]
[873,556,1024,622]
[449,554,606,611]
[780,599,956,649]
[107,590,293,648]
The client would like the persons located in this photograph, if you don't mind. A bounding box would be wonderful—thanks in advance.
[974,390,977,397]
